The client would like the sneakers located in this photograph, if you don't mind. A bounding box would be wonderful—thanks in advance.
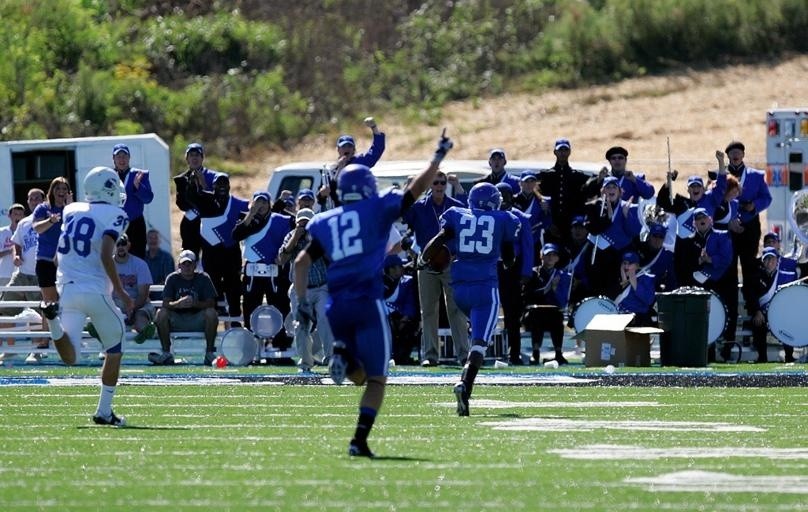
[202,353,215,365]
[529,348,543,364]
[508,355,526,366]
[133,322,156,344]
[419,359,439,368]
[782,354,797,364]
[147,350,175,365]
[87,322,107,361]
[350,436,376,459]
[554,352,571,366]
[328,340,348,388]
[718,349,736,360]
[0,353,20,361]
[40,299,59,320]
[93,408,128,427]
[454,379,471,417]
[25,346,50,363]
[753,352,770,364]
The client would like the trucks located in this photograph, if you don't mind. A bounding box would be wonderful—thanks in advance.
[763,108,807,258]
[264,163,596,214]
[1,132,172,251]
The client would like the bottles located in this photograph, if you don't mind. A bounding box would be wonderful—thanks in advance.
[210,356,227,370]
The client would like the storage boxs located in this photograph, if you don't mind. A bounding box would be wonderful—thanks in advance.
[569,313,666,369]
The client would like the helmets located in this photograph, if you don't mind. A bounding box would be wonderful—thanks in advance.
[83,166,127,209]
[336,162,377,201]
[467,182,504,214]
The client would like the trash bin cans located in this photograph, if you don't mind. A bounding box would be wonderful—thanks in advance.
[655,290,711,367]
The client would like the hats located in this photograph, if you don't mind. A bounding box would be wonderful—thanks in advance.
[649,141,780,263]
[605,147,629,162]
[253,191,272,203]
[538,241,559,257]
[112,143,130,155]
[489,148,506,160]
[520,169,538,181]
[554,138,570,151]
[602,176,621,188]
[295,207,315,222]
[571,215,584,229]
[620,252,640,263]
[179,249,197,265]
[336,135,355,147]
[383,255,407,266]
[185,142,204,155]
[282,187,315,207]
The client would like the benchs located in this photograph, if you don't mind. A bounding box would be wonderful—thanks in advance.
[434,300,791,364]
[0,282,299,365]
